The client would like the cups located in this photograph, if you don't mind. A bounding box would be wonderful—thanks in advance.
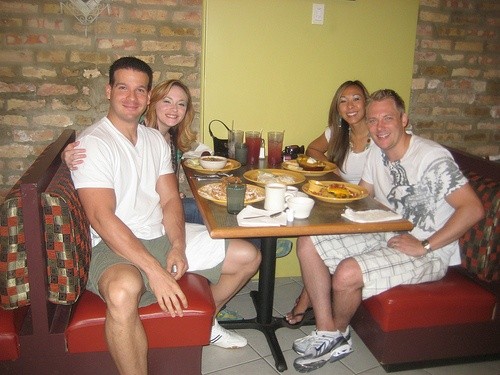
[226,182,247,215]
[245,131,262,163]
[309,180,324,193]
[235,143,248,165]
[268,132,283,166]
[228,130,244,160]
[264,182,315,219]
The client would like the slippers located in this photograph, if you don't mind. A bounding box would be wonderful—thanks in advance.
[282,306,307,329]
[304,308,315,325]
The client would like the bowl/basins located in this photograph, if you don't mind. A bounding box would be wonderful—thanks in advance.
[199,155,227,170]
[297,160,325,171]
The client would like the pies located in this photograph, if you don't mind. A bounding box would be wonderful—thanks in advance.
[327,183,350,195]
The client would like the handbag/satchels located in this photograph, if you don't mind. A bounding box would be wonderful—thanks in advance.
[209,120,235,158]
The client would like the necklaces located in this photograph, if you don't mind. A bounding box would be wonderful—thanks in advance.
[348,128,370,152]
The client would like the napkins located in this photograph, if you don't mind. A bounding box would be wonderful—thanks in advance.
[237,204,288,227]
[341,205,403,223]
[182,143,214,159]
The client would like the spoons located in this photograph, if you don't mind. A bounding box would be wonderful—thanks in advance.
[243,212,282,219]
[187,159,199,166]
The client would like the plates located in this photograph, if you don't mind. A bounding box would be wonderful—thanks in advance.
[280,159,339,177]
[243,169,306,188]
[184,157,241,175]
[197,183,265,206]
[302,181,371,204]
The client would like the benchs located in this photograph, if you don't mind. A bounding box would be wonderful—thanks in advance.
[0,129,215,375]
[350,144,500,372]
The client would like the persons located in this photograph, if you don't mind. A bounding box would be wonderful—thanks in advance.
[283,81,375,329]
[292,89,485,373]
[71,57,262,375]
[61,80,293,320]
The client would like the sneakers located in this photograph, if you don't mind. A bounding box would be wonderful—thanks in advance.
[210,318,247,348]
[293,326,352,356]
[294,330,353,373]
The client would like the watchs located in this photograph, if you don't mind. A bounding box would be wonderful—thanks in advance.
[422,239,431,252]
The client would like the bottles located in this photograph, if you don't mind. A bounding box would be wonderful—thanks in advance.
[259,139,265,159]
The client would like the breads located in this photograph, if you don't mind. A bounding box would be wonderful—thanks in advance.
[285,161,303,171]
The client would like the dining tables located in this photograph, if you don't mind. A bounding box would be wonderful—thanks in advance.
[181,153,414,372]
